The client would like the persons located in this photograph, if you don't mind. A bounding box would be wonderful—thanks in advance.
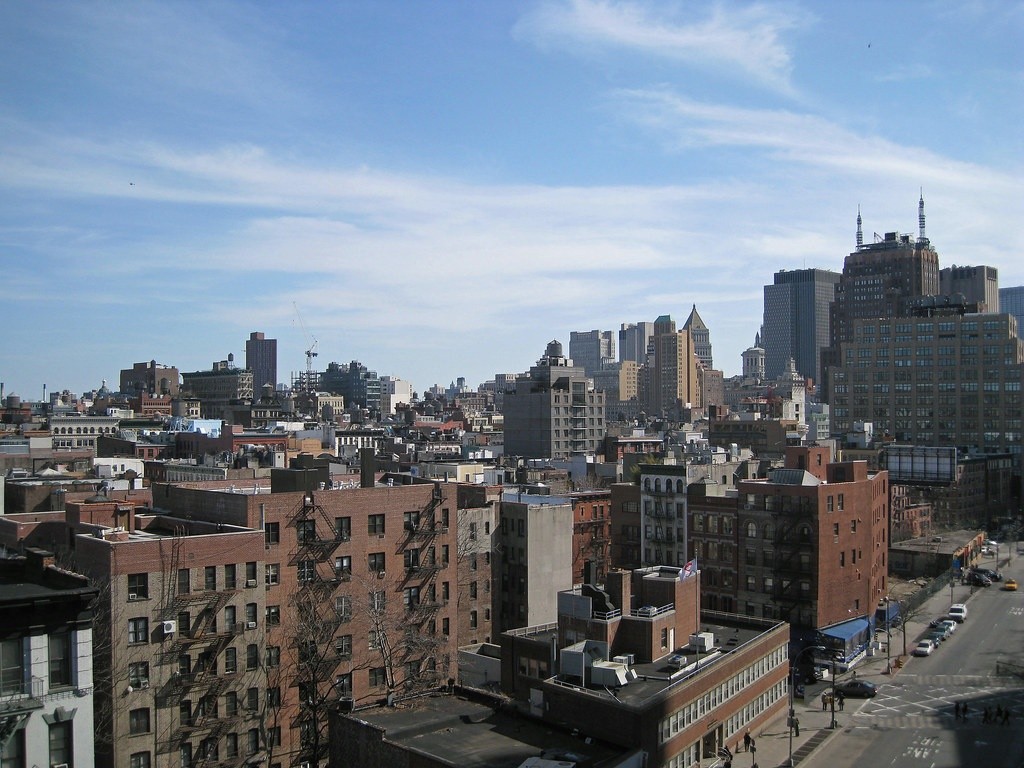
[794,718,800,737]
[837,691,846,711]
[821,691,832,711]
[725,745,730,753]
[851,671,857,681]
[966,573,972,586]
[743,732,751,752]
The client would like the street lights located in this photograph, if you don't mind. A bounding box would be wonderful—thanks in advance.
[787,646,826,767]
[830,649,844,729]
[884,579,914,673]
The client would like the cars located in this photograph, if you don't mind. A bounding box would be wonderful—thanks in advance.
[915,639,934,656]
[832,680,877,698]
[1004,577,1017,590]
[967,573,992,586]
[936,625,950,640]
[927,632,942,648]
[943,620,957,634]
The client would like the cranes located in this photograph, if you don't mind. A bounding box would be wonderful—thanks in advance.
[292,301,319,371]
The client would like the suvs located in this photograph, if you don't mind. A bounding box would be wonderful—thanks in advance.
[949,604,967,623]
[973,568,1001,581]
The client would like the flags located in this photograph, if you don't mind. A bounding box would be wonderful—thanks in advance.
[678,558,697,582]
